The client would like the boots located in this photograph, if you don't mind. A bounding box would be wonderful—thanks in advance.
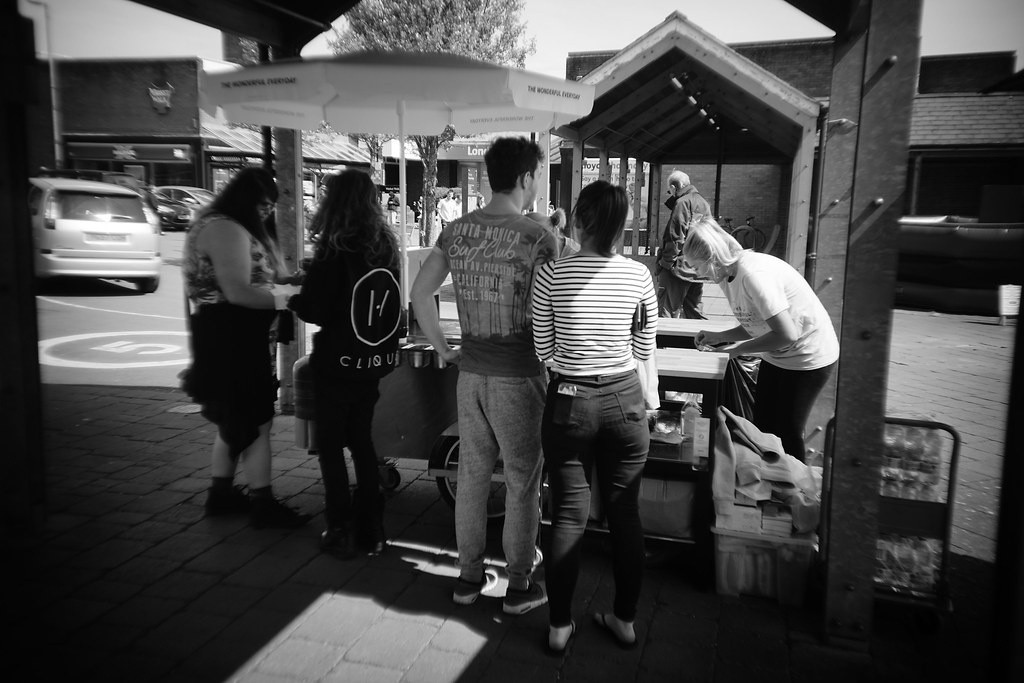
[352,511,386,553]
[319,510,357,559]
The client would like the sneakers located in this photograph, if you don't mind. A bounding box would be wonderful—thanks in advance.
[503,580,549,615]
[453,567,498,604]
[250,497,313,529]
[205,484,251,516]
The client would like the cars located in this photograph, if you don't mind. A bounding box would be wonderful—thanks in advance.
[27,176,161,293]
[153,185,220,229]
[31,166,192,233]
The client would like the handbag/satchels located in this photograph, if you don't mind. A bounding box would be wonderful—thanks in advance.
[275,309,295,345]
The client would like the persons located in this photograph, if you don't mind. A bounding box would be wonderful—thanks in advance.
[524,208,581,257]
[682,216,839,463]
[532,179,657,656]
[386,189,458,231]
[179,167,294,527]
[410,138,559,615]
[287,170,402,559]
[654,172,714,320]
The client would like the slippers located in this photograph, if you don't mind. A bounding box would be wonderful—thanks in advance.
[593,612,638,651]
[542,614,577,657]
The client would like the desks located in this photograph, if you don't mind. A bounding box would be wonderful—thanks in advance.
[534,346,730,592]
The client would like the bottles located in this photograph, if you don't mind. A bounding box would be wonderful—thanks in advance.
[680,392,702,438]
[873,534,942,590]
[879,424,943,502]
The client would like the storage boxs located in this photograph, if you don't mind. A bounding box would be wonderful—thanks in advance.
[637,475,695,539]
[711,525,819,605]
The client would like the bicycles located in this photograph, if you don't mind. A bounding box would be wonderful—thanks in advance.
[720,215,767,251]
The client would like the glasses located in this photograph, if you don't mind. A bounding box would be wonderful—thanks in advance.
[667,183,676,194]
[258,200,277,212]
